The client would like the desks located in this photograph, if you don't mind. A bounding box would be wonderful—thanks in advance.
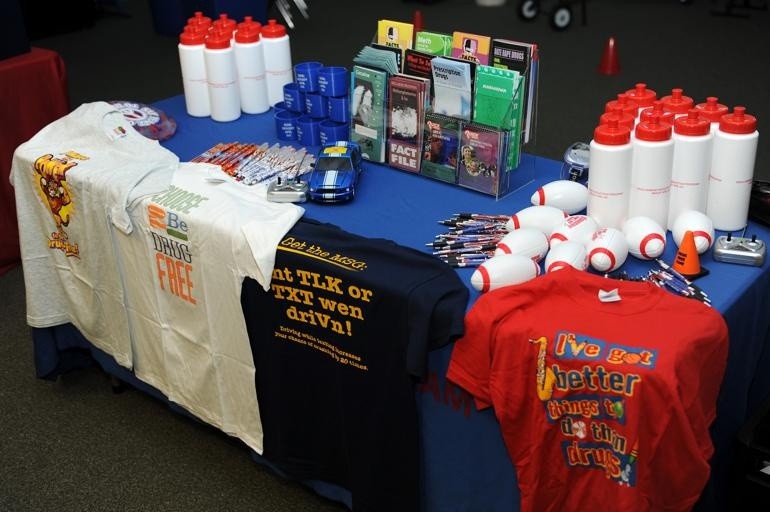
[0,44,71,276]
[10,93,769,512]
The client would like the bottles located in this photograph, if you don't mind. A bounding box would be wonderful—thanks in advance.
[587,80,761,238]
[177,9,294,122]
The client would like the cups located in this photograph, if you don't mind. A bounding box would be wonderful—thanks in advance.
[274,62,349,144]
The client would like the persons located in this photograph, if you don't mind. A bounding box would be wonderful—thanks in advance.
[463,146,496,177]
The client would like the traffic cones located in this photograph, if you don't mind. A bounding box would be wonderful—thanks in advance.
[596,34,622,76]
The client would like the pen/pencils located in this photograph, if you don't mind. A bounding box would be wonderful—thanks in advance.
[424,213,513,268]
[604,258,711,308]
[188,141,317,186]
